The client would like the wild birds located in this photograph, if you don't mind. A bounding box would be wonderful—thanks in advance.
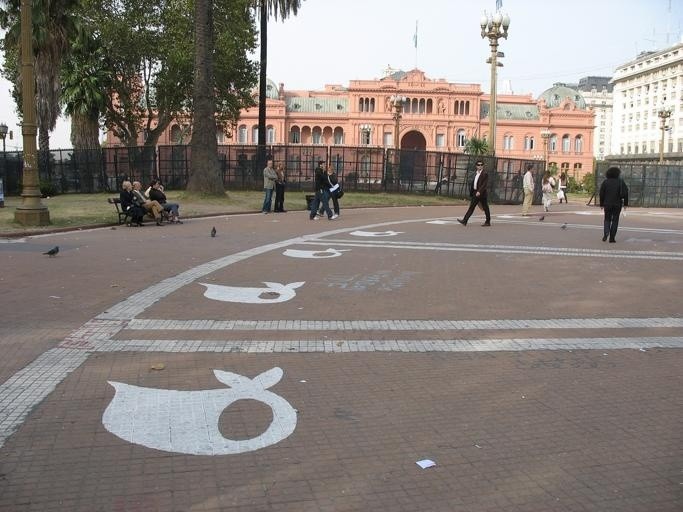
[559,222,567,230]
[210,226,216,237]
[42,246,59,258]
[539,216,545,221]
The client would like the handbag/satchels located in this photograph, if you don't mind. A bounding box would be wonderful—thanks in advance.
[329,184,344,199]
[557,191,564,200]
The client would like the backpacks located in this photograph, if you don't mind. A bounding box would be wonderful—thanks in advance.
[542,177,553,193]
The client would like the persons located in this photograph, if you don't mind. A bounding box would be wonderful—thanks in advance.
[457,160,491,226]
[261,160,278,213]
[519,165,536,215]
[316,166,339,219]
[541,170,556,211]
[510,170,522,201]
[599,167,628,241]
[557,172,568,203]
[273,161,286,213]
[119,177,183,226]
[308,161,335,221]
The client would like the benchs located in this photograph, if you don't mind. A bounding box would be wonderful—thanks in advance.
[108,198,179,227]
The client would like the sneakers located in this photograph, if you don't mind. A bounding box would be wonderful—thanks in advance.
[313,212,338,220]
[603,235,616,243]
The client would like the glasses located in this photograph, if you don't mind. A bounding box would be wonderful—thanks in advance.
[477,164,483,166]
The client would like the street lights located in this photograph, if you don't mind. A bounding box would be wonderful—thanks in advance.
[0,122,12,159]
[476,1,510,173]
[532,154,542,161]
[658,97,674,162]
[359,123,372,181]
[386,95,408,174]
[539,132,555,168]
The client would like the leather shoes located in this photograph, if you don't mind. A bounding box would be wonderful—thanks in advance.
[457,219,467,226]
[157,210,183,226]
[130,221,144,227]
[481,223,491,226]
